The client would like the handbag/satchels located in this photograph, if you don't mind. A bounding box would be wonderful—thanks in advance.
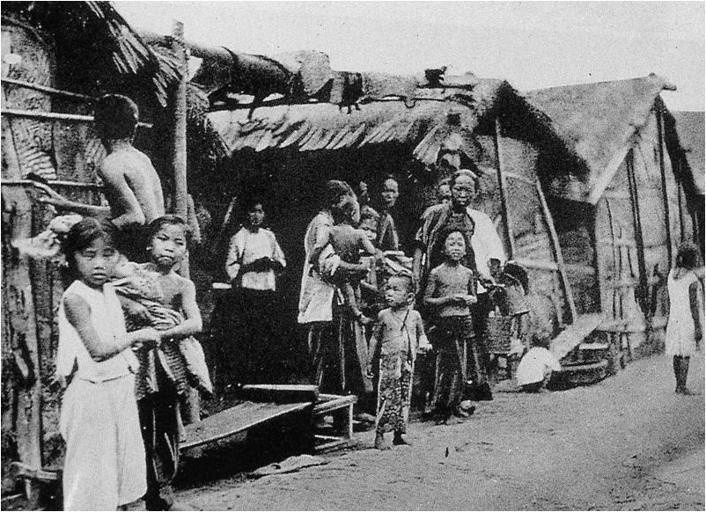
[483,305,512,354]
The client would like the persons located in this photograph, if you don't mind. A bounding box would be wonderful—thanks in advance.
[298,165,533,451]
[518,331,561,391]
[56,215,148,511]
[34,93,165,261]
[113,216,203,511]
[224,195,288,372]
[664,241,704,395]
